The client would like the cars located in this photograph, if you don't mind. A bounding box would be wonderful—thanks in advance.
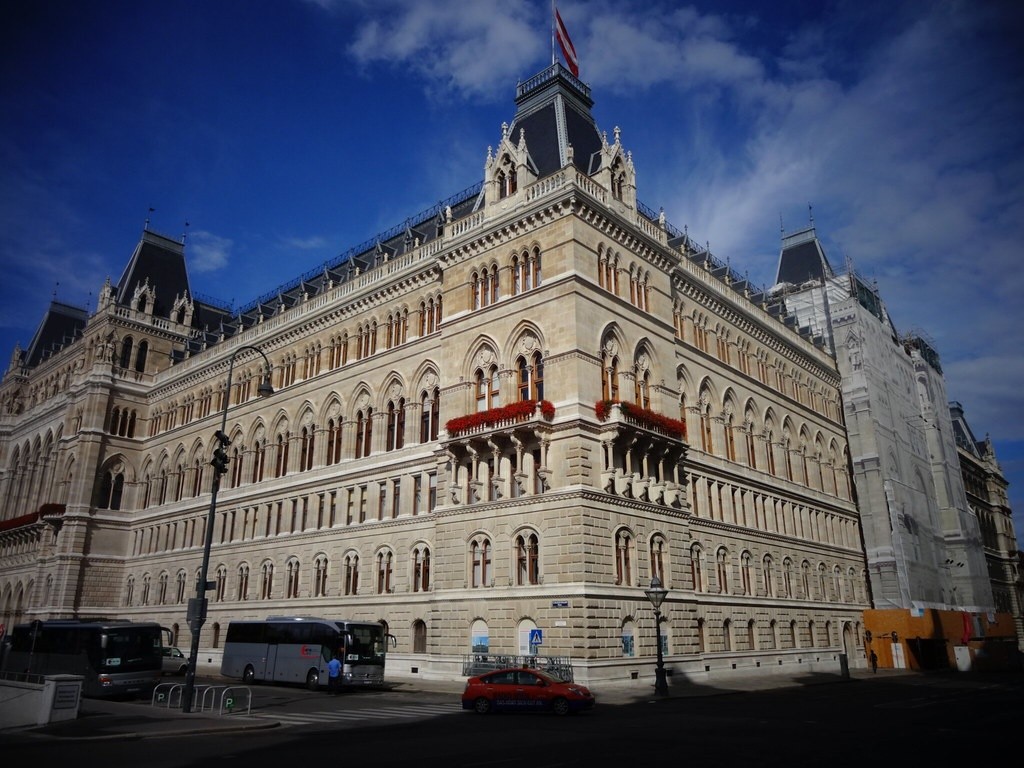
[461,664,598,718]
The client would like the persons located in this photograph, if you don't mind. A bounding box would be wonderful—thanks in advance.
[326,656,342,695]
[869,650,878,674]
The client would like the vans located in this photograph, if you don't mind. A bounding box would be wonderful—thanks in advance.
[153,645,190,678]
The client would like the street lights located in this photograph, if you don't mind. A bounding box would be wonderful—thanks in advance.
[181,345,277,710]
[643,573,672,700]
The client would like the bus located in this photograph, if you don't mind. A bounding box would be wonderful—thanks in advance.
[220,615,397,694]
[1,617,174,700]
